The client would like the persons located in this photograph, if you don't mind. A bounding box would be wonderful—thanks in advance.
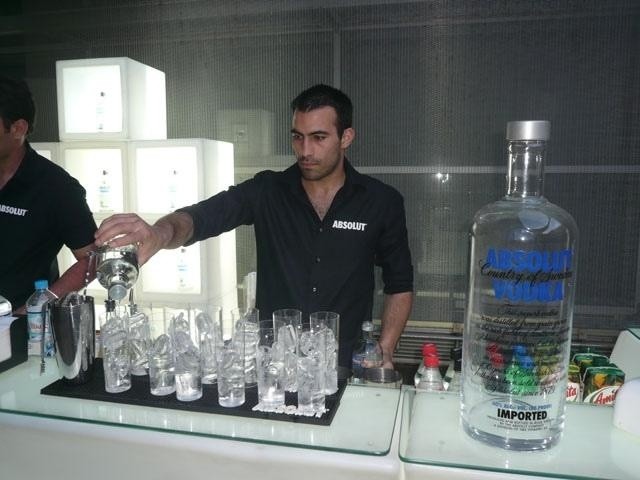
[0,68,104,318]
[93,85,414,374]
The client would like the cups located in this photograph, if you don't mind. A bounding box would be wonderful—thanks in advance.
[94,303,340,410]
[25,279,57,358]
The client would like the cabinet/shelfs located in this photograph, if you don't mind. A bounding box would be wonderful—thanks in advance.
[55,57,169,142]
[29,142,238,341]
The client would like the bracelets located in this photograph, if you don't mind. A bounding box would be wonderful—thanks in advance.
[46,288,60,300]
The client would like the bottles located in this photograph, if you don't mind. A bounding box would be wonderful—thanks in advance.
[462,117,580,456]
[411,338,464,394]
[95,234,140,302]
[351,321,384,371]
